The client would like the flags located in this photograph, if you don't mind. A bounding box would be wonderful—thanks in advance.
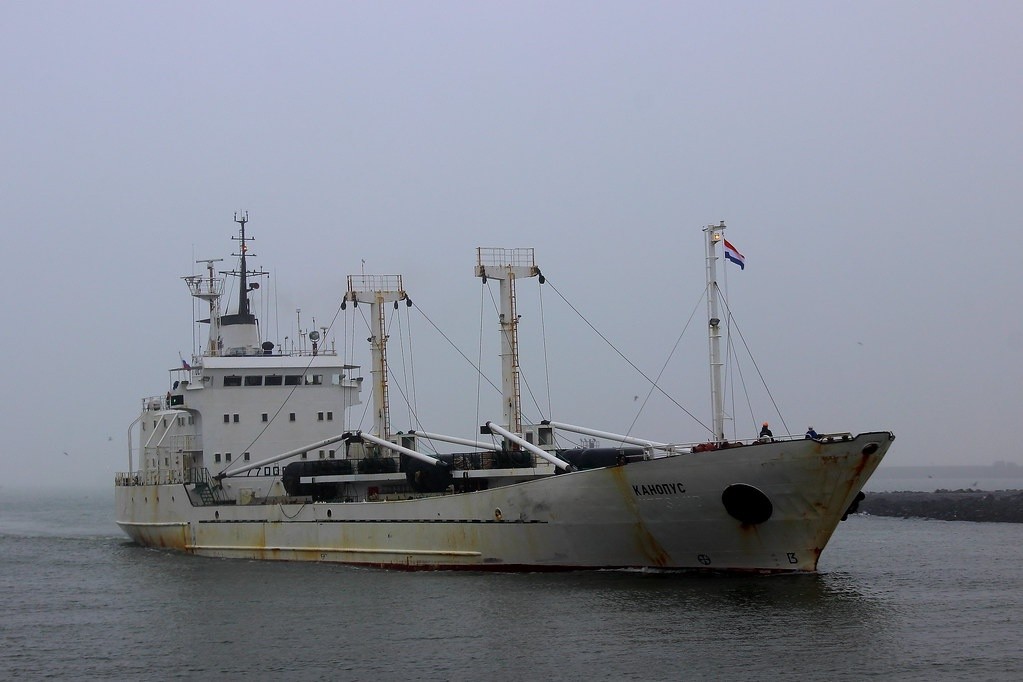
[724,238,745,270]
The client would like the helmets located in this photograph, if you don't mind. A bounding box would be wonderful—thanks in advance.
[763,422,769,427]
[808,424,814,430]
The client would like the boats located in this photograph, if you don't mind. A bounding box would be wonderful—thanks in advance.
[115,207,896,579]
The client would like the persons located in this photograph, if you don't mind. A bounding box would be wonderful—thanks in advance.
[805,424,819,440]
[759,422,774,441]
